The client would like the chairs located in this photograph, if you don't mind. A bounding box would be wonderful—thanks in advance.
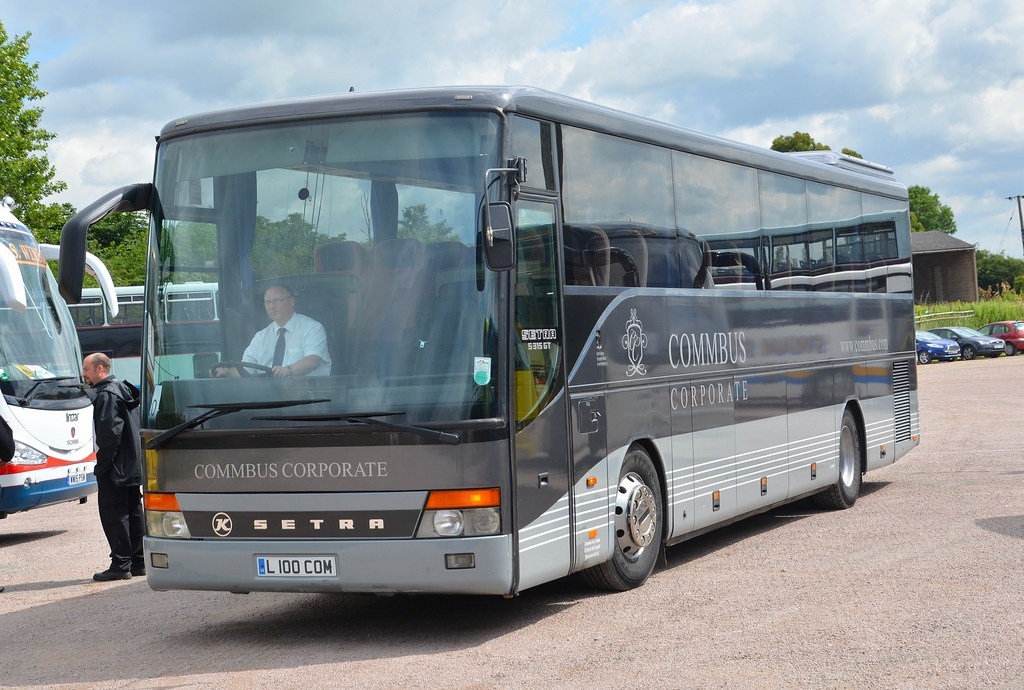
[315,223,898,372]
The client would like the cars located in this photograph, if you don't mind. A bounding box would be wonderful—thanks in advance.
[915,330,961,364]
[976,320,1024,356]
[926,326,1007,360]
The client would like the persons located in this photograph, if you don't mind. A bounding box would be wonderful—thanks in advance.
[82,352,148,582]
[208,282,333,378]
[0,415,15,467]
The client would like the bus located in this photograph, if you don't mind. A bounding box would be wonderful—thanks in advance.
[57,84,919,600]
[64,276,224,388]
[510,221,775,459]
[0,203,121,520]
[693,207,918,409]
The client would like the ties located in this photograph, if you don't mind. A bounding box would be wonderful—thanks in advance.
[271,328,287,368]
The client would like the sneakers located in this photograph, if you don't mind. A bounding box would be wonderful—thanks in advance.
[93,568,132,581]
[132,568,146,575]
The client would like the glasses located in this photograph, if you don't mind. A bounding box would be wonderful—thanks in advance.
[264,296,291,304]
